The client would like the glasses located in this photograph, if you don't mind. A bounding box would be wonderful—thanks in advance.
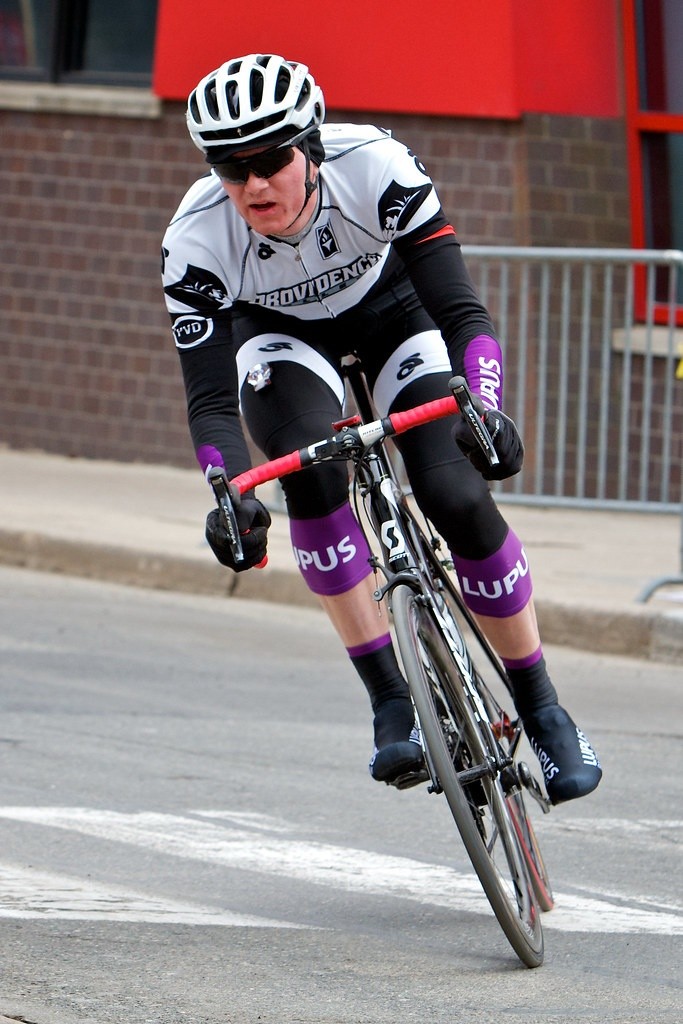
[209,123,320,185]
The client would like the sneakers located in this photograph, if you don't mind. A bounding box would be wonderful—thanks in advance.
[522,703,603,801]
[367,697,425,783]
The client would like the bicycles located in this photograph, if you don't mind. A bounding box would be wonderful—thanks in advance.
[195,376,559,969]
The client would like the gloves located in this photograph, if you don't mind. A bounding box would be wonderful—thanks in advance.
[206,497,271,574]
[452,408,526,481]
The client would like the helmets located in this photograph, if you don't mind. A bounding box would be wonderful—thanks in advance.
[184,51,326,154]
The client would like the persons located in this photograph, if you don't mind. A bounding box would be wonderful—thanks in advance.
[161,51,601,804]
